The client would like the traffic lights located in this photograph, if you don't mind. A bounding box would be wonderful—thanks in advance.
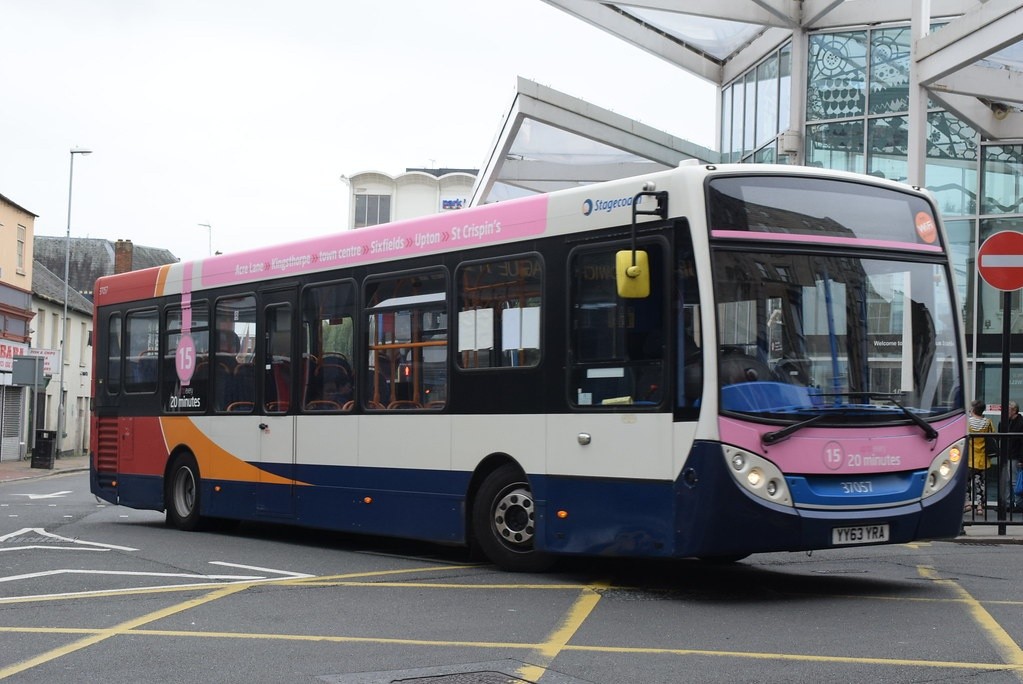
[425,387,431,399]
[398,364,412,381]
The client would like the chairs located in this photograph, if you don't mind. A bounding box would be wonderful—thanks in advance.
[108,345,538,414]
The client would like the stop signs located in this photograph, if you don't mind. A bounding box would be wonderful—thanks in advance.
[977,230,1023,292]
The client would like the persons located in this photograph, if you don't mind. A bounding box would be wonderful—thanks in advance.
[963,399,995,516]
[993,401,1023,513]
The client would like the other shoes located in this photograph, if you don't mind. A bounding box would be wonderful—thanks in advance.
[994,502,1022,513]
[963,504,971,513]
[976,506,983,515]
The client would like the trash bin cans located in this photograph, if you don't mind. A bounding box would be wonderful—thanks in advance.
[31,429,57,469]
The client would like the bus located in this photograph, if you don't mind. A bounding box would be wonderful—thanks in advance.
[88,160,970,574]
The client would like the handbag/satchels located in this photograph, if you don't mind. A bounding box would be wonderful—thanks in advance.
[985,436,999,457]
[1014,467,1023,495]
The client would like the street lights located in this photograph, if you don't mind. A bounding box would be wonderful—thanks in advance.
[198,223,212,256]
[56,148,92,460]
